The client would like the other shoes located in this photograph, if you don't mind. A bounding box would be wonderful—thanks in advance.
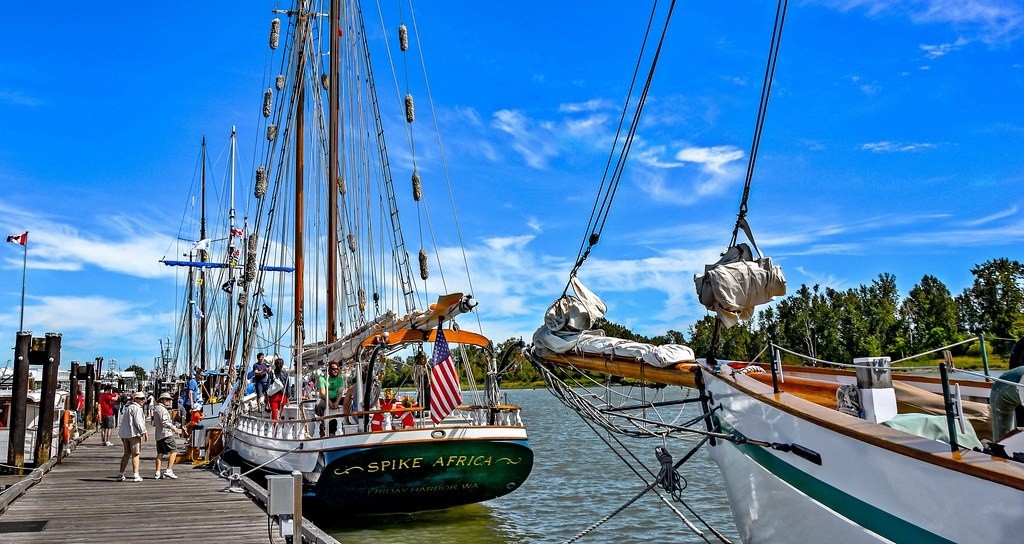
[106,442,113,446]
[133,476,143,481]
[154,474,166,479]
[117,475,125,481]
[101,442,105,446]
[164,471,178,479]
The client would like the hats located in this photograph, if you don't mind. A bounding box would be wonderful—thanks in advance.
[157,393,174,403]
[192,403,203,412]
[134,392,146,398]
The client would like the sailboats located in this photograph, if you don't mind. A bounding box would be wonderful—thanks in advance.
[146,0,531,527]
[521,0,1024,544]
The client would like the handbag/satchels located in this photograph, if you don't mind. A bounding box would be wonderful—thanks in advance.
[267,370,284,396]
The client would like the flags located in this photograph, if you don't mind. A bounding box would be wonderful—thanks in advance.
[195,239,211,252]
[6,233,27,246]
[429,325,463,425]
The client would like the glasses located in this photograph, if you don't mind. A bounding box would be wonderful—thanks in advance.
[331,368,338,370]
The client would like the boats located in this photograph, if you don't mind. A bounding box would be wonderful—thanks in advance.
[0,359,73,467]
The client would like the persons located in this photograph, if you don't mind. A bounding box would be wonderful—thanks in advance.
[154,393,178,479]
[99,352,381,446]
[117,391,149,481]
[76,390,84,423]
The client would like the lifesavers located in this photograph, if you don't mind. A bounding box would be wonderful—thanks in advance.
[97,404,101,424]
[62,409,70,447]
